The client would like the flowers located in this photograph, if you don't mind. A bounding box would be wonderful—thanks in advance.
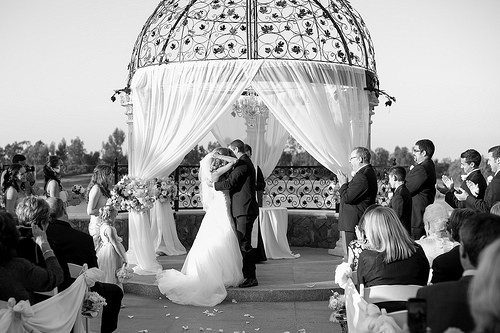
[328,289,345,323]
[410,165,414,170]
[351,171,356,177]
[81,291,107,319]
[8,168,12,174]
[486,175,493,186]
[110,87,130,102]
[461,175,469,181]
[47,162,52,168]
[71,183,86,196]
[364,86,396,107]
[106,175,178,214]
[116,266,134,279]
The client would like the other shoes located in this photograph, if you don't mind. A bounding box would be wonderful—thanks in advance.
[120,304,126,308]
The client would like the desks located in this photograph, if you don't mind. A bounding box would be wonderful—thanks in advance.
[259,207,301,259]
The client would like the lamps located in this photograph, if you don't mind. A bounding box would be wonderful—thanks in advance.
[231,85,270,127]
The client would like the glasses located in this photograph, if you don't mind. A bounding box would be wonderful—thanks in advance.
[414,149,422,153]
[349,156,361,160]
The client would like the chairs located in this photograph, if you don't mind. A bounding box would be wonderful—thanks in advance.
[351,271,425,329]
[33,263,89,333]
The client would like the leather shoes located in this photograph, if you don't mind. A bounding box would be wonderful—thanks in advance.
[241,279,258,287]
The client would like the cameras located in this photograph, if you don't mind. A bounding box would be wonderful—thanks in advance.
[20,225,34,238]
[26,164,34,172]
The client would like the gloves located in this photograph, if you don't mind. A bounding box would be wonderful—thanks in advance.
[29,224,53,255]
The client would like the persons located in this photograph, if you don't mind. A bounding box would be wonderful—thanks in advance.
[97,205,129,306]
[0,155,123,333]
[85,163,113,250]
[207,139,265,288]
[156,147,245,308]
[337,146,378,258]
[347,139,500,333]
